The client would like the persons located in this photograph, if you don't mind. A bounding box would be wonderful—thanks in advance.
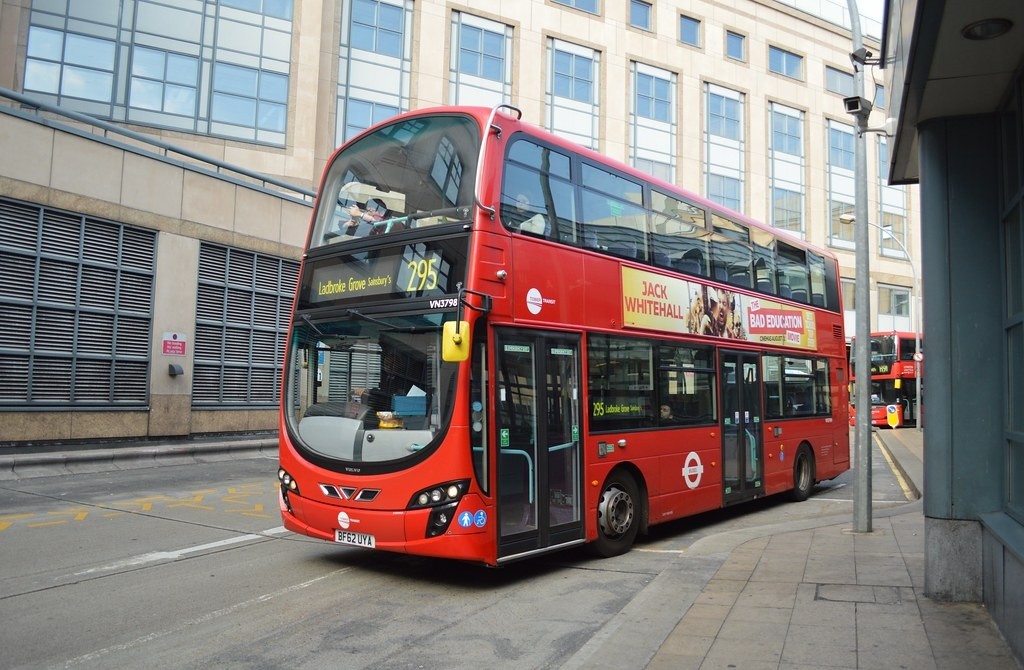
[353,354,406,410]
[341,199,404,234]
[516,192,552,236]
[699,290,736,338]
[661,403,673,419]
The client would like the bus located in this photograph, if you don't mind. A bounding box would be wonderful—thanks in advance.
[848,330,925,428]
[274,103,851,570]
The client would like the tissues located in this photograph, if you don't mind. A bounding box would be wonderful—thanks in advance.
[391,384,428,415]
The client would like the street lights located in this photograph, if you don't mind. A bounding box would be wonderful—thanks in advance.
[837,214,922,433]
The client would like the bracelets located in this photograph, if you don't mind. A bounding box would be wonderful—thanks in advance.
[361,214,365,218]
